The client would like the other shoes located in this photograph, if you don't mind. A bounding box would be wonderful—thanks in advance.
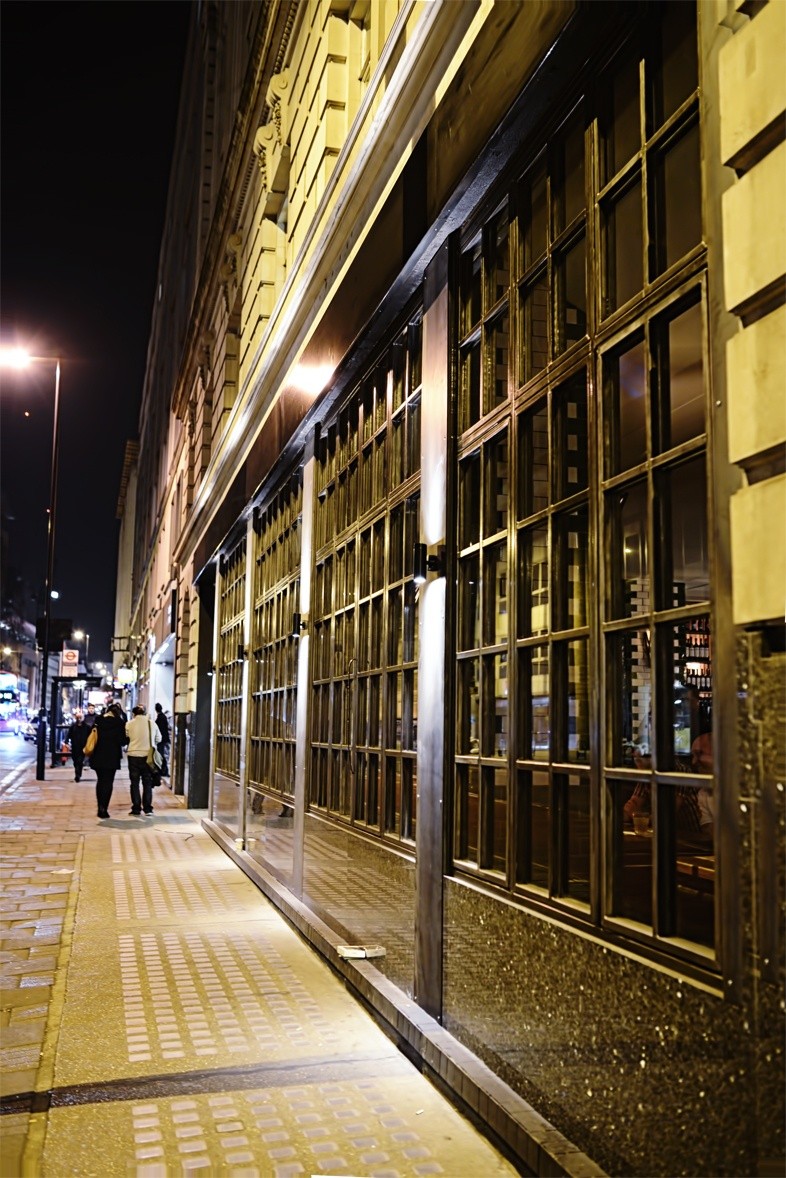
[145,811,154,816]
[98,810,110,818]
[128,812,140,816]
[75,777,80,783]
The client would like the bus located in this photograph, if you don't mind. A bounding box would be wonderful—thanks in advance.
[0,688,23,736]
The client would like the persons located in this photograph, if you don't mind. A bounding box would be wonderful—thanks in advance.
[125,707,162,816]
[89,705,129,818]
[154,703,171,778]
[65,702,146,783]
[622,684,716,837]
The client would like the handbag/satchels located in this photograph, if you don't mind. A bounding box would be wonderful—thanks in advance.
[82,714,102,756]
[146,747,163,772]
[62,744,69,760]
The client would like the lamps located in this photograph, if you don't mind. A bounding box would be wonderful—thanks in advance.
[414,543,449,583]
[207,661,215,675]
[237,645,247,662]
[293,614,306,637]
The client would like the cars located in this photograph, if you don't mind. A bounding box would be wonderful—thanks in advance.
[23,712,76,741]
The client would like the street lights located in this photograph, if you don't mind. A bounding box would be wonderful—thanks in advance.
[75,631,89,666]
[1,343,63,781]
